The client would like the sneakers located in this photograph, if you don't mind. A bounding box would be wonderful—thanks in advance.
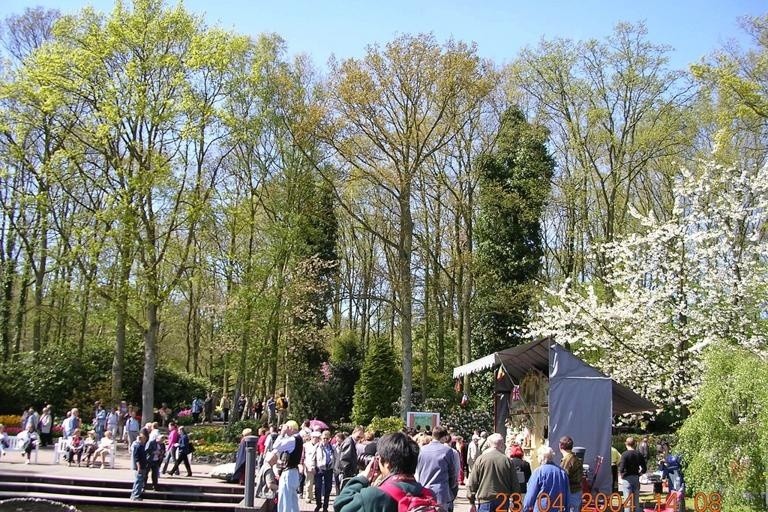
[21,451,26,458]
[25,462,31,465]
[152,487,161,493]
[296,492,333,512]
[65,459,109,471]
[130,495,144,502]
[158,473,194,478]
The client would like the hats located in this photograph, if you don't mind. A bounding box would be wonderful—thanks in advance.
[86,430,97,437]
[472,435,481,440]
[309,431,322,438]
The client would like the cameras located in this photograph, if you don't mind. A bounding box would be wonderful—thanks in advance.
[357,453,380,471]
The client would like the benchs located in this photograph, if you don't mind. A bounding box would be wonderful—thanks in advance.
[53,435,118,469]
[0,433,42,463]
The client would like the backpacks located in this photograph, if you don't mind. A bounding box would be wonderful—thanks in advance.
[507,458,527,486]
[376,481,443,512]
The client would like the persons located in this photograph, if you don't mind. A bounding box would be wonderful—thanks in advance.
[1,393,686,512]
